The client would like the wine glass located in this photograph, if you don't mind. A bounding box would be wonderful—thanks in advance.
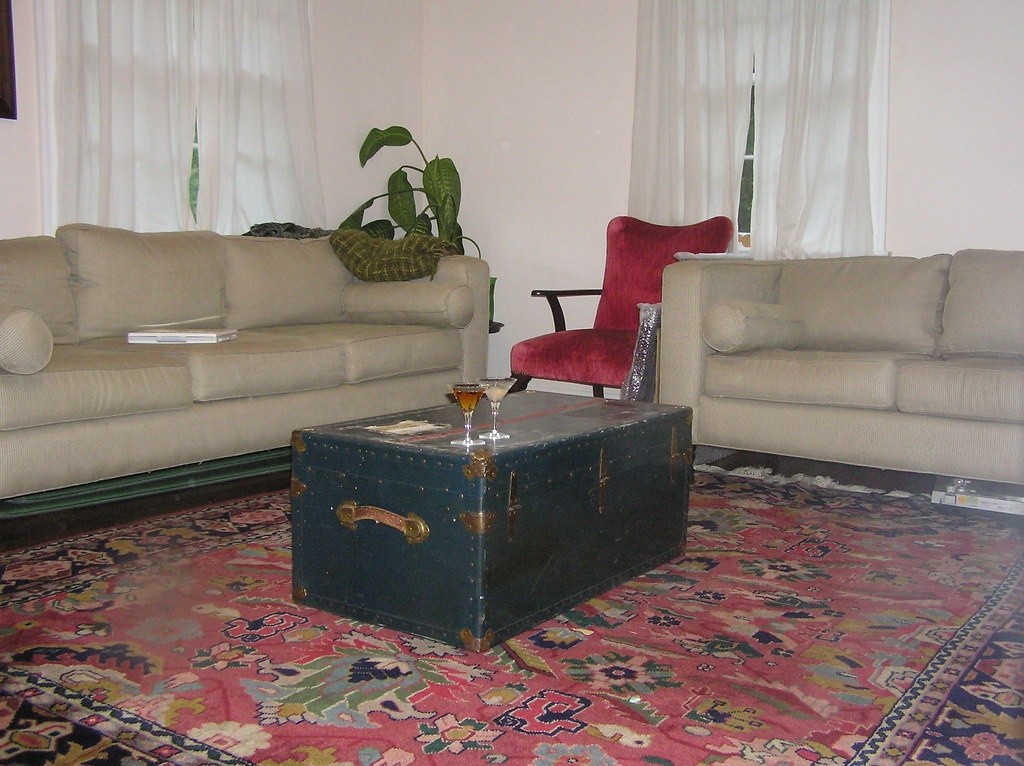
[475,377,518,440]
[447,383,488,445]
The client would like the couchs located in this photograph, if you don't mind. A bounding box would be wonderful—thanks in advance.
[0,222,490,552]
[658,248,1024,486]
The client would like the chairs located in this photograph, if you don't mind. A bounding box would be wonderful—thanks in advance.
[506,216,736,398]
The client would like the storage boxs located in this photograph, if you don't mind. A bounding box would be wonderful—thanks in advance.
[932,476,1024,515]
[291,390,692,653]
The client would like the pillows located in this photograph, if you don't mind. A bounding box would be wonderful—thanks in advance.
[701,300,806,354]
[0,305,53,373]
[343,280,475,328]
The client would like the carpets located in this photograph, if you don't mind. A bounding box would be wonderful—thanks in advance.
[0,463,1024,766]
